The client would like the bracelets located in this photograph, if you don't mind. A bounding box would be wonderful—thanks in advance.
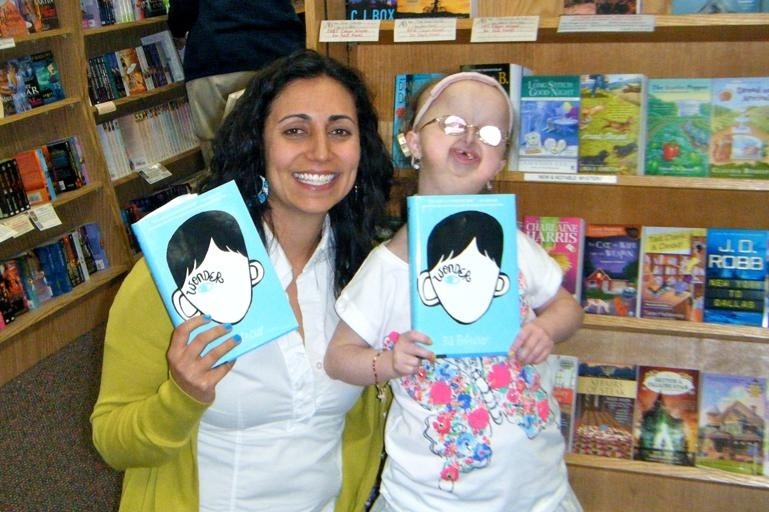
[371,348,392,401]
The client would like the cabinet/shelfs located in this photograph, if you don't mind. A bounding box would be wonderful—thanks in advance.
[306,0,767,512]
[1,1,210,387]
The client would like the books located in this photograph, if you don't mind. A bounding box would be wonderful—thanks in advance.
[405,193,522,355]
[537,355,769,478]
[130,179,299,368]
[520,215,769,330]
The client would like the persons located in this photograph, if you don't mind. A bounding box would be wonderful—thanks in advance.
[167,0,306,172]
[321,69,586,512]
[87,46,392,511]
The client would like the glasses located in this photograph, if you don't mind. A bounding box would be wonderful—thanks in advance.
[418,115,510,147]
